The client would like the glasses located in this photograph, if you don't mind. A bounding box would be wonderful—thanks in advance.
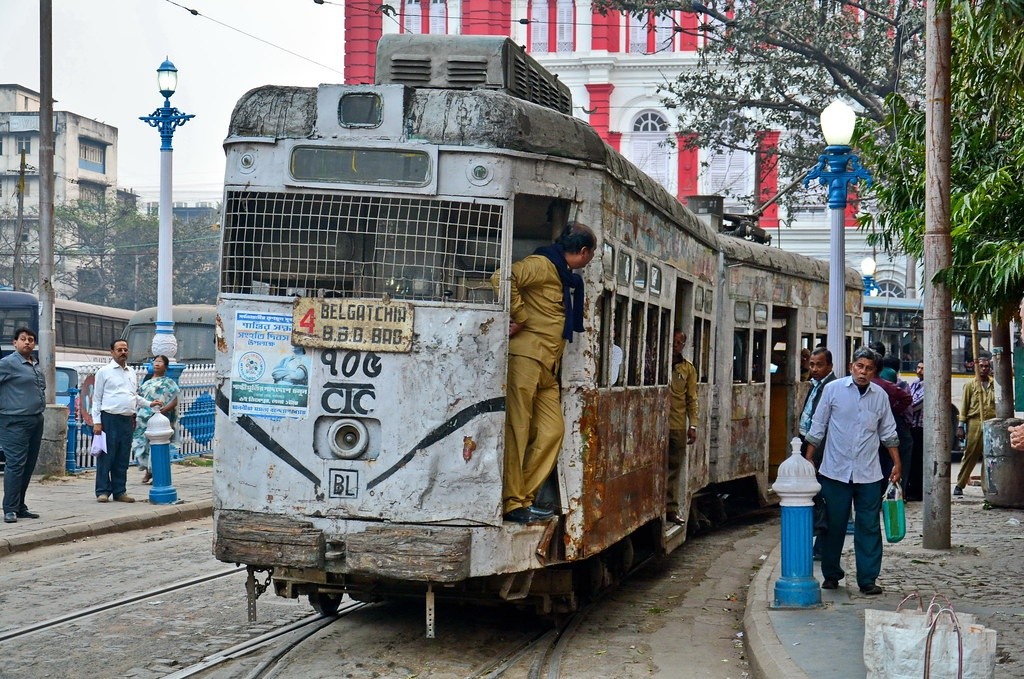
[112,348,128,352]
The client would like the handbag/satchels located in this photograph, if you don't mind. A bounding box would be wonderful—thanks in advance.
[881,479,906,542]
[863,592,996,679]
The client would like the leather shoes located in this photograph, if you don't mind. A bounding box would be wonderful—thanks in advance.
[666,512,684,523]
[504,507,542,523]
[526,504,554,519]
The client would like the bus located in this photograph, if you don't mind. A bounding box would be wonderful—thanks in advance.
[863,296,1024,453]
[0,289,136,425]
[211,36,864,625]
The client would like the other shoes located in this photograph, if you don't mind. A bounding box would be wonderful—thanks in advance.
[859,584,882,593]
[98,494,108,502]
[4,511,17,522]
[18,512,39,518]
[845,520,856,533]
[113,494,136,502]
[954,487,964,495]
[142,473,152,482]
[822,578,839,589]
[145,480,153,485]
[813,554,822,561]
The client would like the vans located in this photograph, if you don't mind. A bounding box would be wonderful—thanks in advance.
[119,303,220,396]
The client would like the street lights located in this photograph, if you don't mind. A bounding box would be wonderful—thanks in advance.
[861,255,877,347]
[803,92,874,377]
[139,56,195,462]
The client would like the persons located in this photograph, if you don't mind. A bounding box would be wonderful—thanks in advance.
[491,224,597,522]
[871,341,925,502]
[798,348,838,560]
[965,336,984,361]
[903,316,923,361]
[132,355,179,485]
[806,348,901,594]
[1008,299,1024,451]
[91,338,162,502]
[802,348,811,381]
[667,329,697,523]
[611,326,622,385]
[954,359,996,494]
[0,326,46,522]
